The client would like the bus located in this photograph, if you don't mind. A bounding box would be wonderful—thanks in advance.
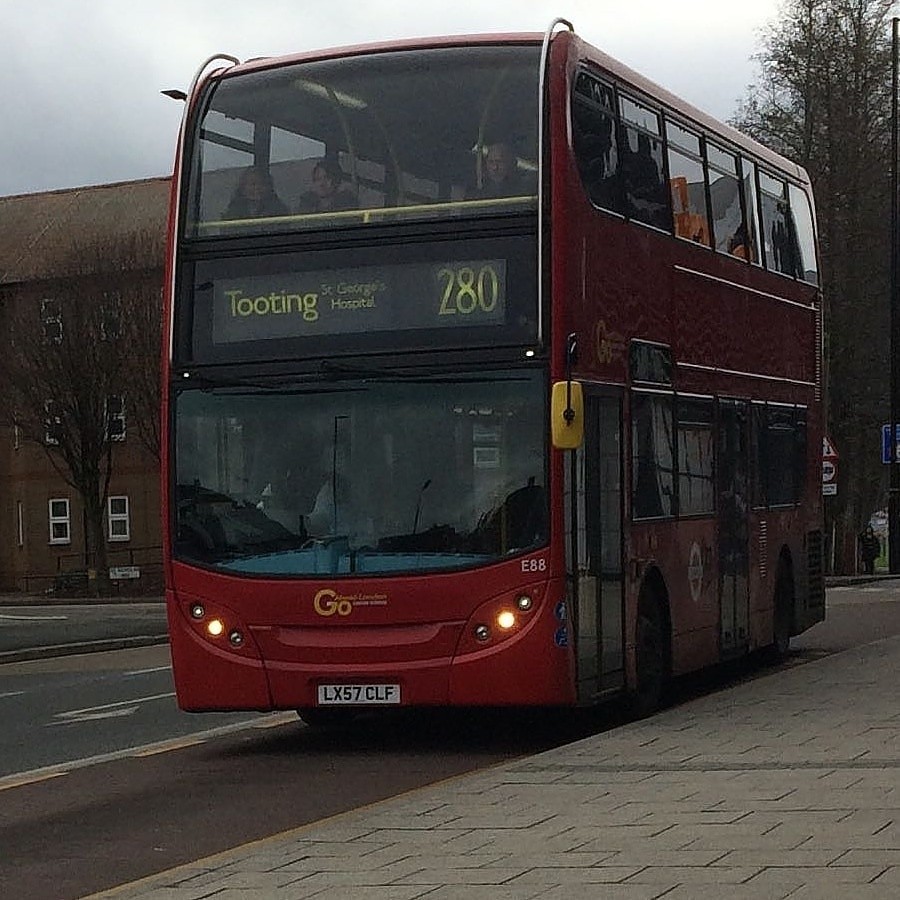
[160,18,829,732]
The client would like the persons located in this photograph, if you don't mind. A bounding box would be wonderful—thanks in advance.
[860,527,881,574]
[460,142,538,216]
[298,160,360,229]
[221,165,289,232]
